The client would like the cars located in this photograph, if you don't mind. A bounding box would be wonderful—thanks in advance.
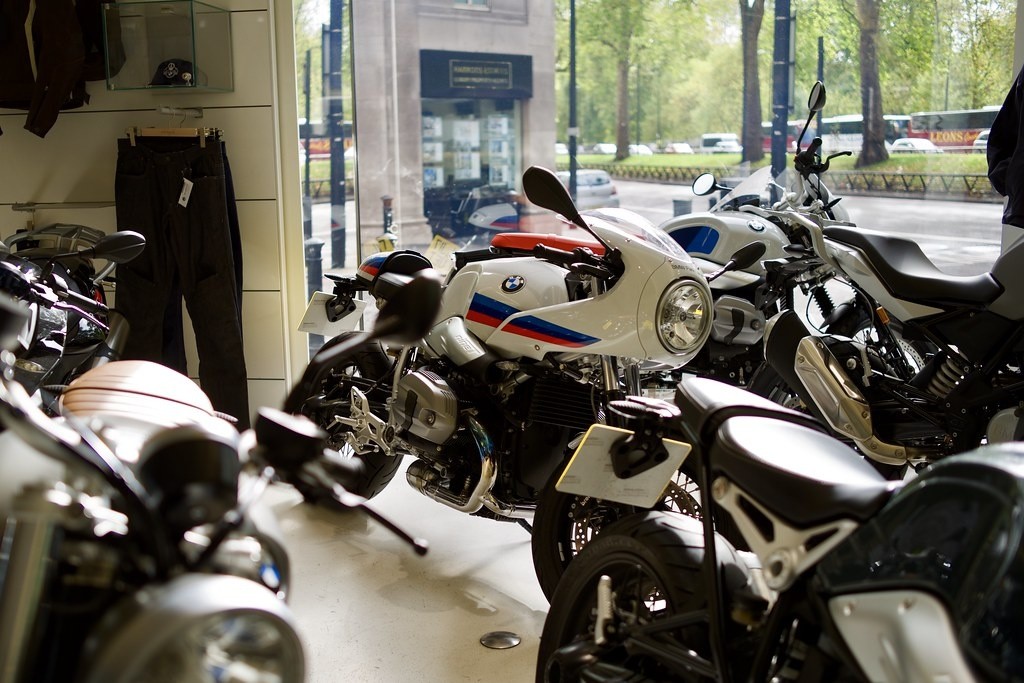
[591,142,617,155]
[891,137,940,155]
[712,142,743,154]
[628,144,653,155]
[971,129,992,155]
[557,169,620,214]
[664,142,694,155]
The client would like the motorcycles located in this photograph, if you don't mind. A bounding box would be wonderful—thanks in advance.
[282,160,770,612]
[528,365,1024,683]
[658,81,1024,481]
[1,210,311,683]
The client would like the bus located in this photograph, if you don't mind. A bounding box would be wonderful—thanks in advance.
[822,112,911,155]
[761,117,819,155]
[906,103,1004,154]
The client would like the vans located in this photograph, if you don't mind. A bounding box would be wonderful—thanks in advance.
[700,132,741,153]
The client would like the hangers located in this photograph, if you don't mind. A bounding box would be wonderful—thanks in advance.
[124,106,221,148]
